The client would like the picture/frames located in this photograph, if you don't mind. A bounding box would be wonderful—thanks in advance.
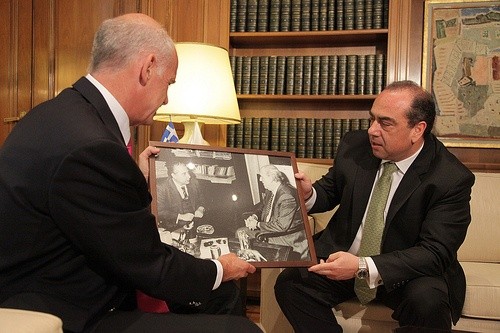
[149,141,317,267]
[421,0,500,148]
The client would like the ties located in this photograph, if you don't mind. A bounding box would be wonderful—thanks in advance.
[126,136,169,314]
[354,162,399,305]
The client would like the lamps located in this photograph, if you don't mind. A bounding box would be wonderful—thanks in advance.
[152,42,242,146]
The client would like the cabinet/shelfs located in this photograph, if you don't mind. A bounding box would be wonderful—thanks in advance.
[229,29,389,164]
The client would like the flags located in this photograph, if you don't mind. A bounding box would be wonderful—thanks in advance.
[161,122,179,143]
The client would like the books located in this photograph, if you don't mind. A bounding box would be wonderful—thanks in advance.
[226,117,371,159]
[230,0,388,32]
[230,54,385,96]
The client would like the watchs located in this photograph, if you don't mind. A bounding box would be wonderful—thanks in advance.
[355,257,368,280]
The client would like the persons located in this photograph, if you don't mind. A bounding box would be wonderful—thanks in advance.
[157,159,206,233]
[236,164,309,259]
[273,80,475,333]
[0,13,265,333]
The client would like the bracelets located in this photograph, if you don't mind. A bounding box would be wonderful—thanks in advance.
[256,222,260,229]
[305,188,313,201]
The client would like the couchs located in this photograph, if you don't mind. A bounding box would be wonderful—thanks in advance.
[296,162,500,333]
